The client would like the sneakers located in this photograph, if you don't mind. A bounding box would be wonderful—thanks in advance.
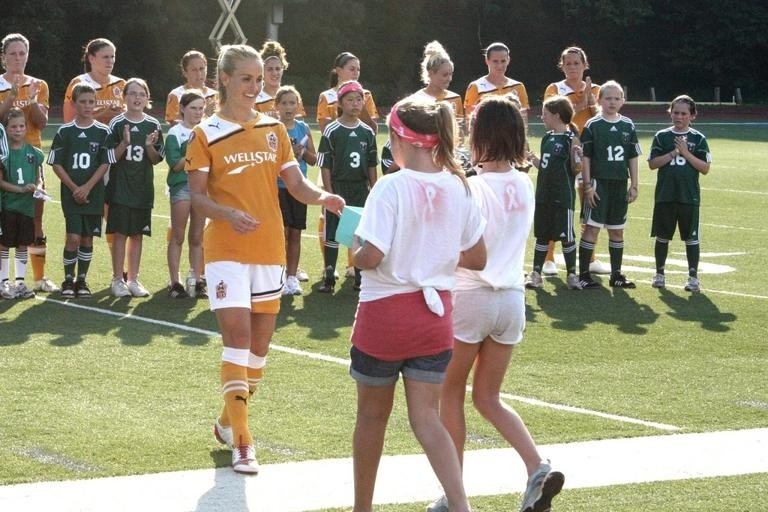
[524,259,636,289]
[427,496,450,512]
[685,277,700,292]
[0,273,209,299]
[230,443,258,474]
[652,273,665,287]
[281,265,362,296]
[519,459,564,512]
[214,414,234,452]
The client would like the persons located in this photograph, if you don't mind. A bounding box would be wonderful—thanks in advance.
[349,93,490,512]
[184,42,348,477]
[0,32,377,303]
[427,94,565,512]
[387,40,711,288]
